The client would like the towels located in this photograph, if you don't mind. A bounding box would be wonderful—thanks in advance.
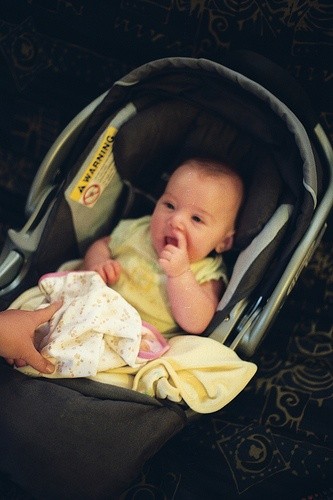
[5,270,259,415]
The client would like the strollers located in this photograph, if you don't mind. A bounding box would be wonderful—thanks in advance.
[0,54,333,498]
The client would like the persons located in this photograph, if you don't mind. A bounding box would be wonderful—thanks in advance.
[30,152,246,380]
[1,299,68,376]
